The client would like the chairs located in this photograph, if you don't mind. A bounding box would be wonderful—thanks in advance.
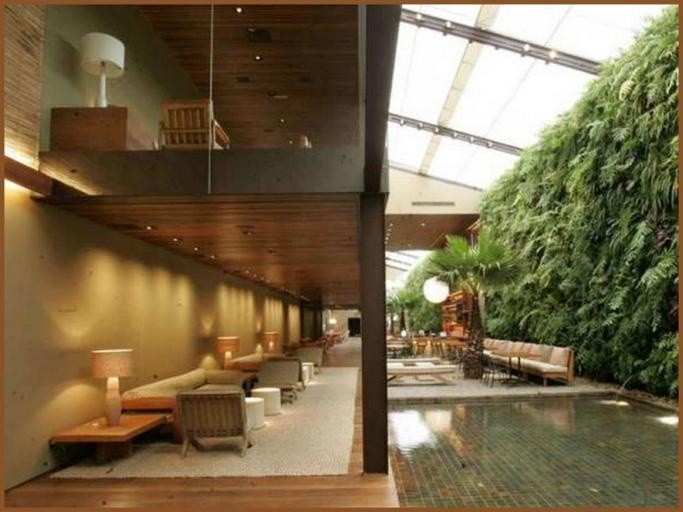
[161,100,230,150]
[174,392,255,459]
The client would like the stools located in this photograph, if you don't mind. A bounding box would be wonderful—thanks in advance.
[243,397,266,430]
[251,388,281,415]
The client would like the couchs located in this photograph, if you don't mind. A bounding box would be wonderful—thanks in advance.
[389,326,576,390]
[123,369,259,434]
[216,328,351,405]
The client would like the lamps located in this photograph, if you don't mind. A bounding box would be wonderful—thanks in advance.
[424,277,450,303]
[77,32,127,107]
[89,349,135,428]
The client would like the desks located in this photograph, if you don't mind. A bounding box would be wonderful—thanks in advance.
[49,413,173,465]
[50,106,153,151]
